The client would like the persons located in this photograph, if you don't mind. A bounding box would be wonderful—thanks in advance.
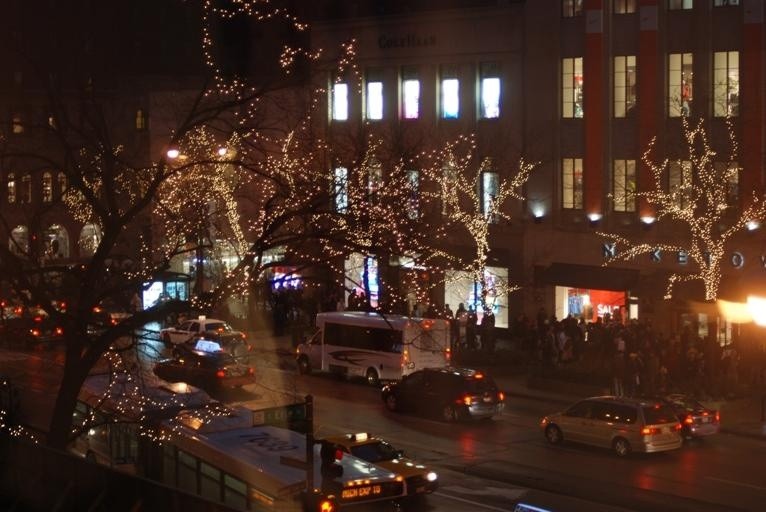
[450,304,766,398]
[130,292,144,315]
[157,291,173,306]
[176,312,187,327]
[261,279,455,336]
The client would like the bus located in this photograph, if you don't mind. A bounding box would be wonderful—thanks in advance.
[69,391,408,512]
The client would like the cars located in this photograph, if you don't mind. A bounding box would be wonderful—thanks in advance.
[320,431,438,496]
[0,297,119,343]
[153,318,254,393]
[379,365,505,422]
[540,390,722,459]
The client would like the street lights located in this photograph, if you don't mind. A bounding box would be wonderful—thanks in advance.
[165,145,227,307]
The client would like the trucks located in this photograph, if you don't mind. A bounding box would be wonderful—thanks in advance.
[295,310,451,387]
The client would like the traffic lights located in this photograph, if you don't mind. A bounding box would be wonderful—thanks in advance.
[311,442,344,512]
